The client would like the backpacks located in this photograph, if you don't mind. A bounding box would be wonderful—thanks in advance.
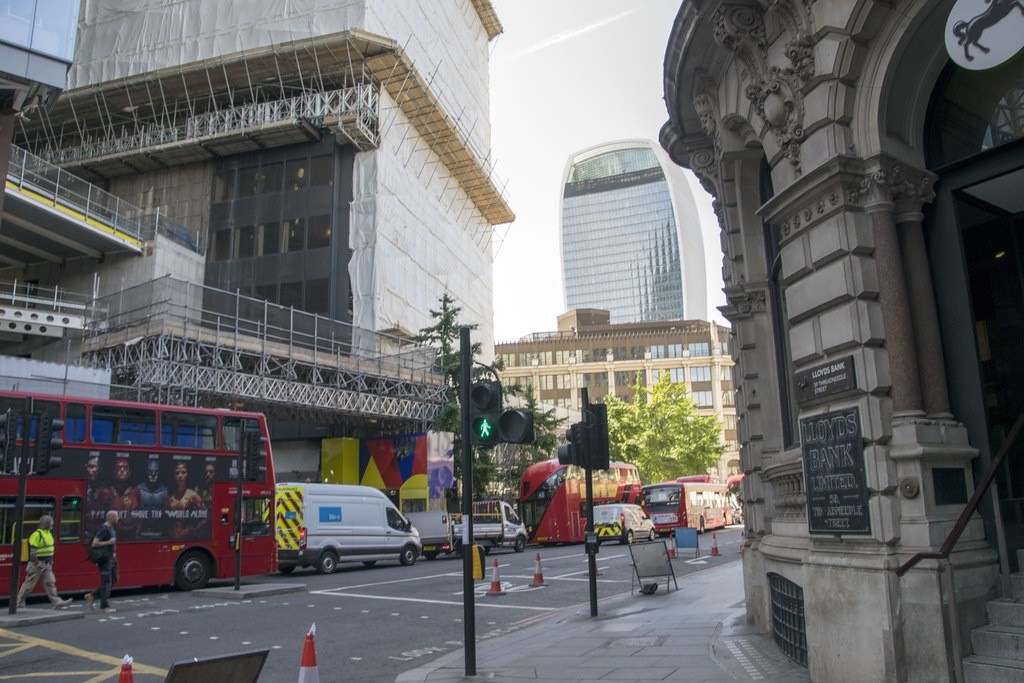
[88,526,111,566]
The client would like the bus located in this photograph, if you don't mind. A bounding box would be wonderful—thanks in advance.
[0,389,279,601]
[519,458,649,544]
[642,476,743,537]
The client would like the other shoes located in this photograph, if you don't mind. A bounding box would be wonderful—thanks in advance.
[84,593,95,611]
[56,598,73,609]
[100,607,117,615]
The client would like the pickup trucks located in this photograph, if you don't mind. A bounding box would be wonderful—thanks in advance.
[453,500,530,553]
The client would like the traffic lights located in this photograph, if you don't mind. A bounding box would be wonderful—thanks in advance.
[35,411,65,473]
[558,423,583,469]
[0,408,20,472]
[499,410,535,443]
[470,381,502,445]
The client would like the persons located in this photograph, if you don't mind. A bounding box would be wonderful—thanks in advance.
[17,515,72,610]
[85,510,119,614]
[85,455,216,542]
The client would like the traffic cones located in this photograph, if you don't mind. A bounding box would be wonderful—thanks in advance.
[485,558,507,596]
[298,633,319,683]
[668,534,676,558]
[528,552,548,587]
[118,654,133,683]
[708,532,721,556]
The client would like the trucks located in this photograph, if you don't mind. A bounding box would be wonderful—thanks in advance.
[400,473,463,559]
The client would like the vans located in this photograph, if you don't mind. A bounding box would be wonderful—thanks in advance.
[276,483,422,574]
[593,503,656,543]
